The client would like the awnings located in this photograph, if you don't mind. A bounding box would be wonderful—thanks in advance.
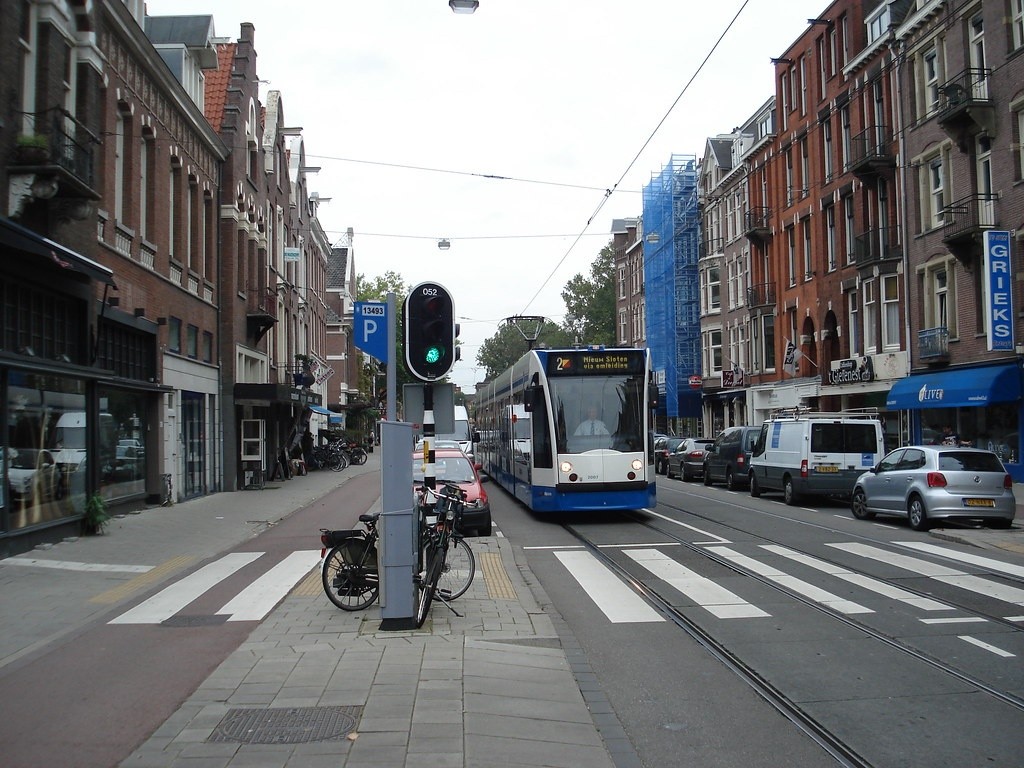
[886,364,1020,410]
[0,215,118,290]
[309,406,343,423]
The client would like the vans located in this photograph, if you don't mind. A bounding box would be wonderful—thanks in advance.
[701,425,763,491]
[45,411,121,492]
[418,405,481,467]
[748,406,888,505]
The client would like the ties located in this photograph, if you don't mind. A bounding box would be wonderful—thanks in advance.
[590,422,595,436]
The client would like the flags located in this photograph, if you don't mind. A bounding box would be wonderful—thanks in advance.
[732,364,742,384]
[782,340,803,376]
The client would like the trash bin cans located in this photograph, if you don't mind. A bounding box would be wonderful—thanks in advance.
[369,446,373,453]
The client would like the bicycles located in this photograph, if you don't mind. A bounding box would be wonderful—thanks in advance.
[415,485,479,629]
[305,436,369,473]
[317,496,478,612]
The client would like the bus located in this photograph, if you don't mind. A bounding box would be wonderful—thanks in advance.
[469,314,661,514]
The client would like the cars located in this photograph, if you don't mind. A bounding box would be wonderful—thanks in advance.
[663,437,717,482]
[0,444,64,508]
[653,433,687,474]
[114,439,145,477]
[410,439,492,537]
[850,444,1018,531]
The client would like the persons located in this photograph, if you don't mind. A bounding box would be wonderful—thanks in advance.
[370,429,374,437]
[933,422,971,446]
[574,405,610,435]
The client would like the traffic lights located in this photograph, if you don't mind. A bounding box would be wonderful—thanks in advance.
[405,281,456,383]
